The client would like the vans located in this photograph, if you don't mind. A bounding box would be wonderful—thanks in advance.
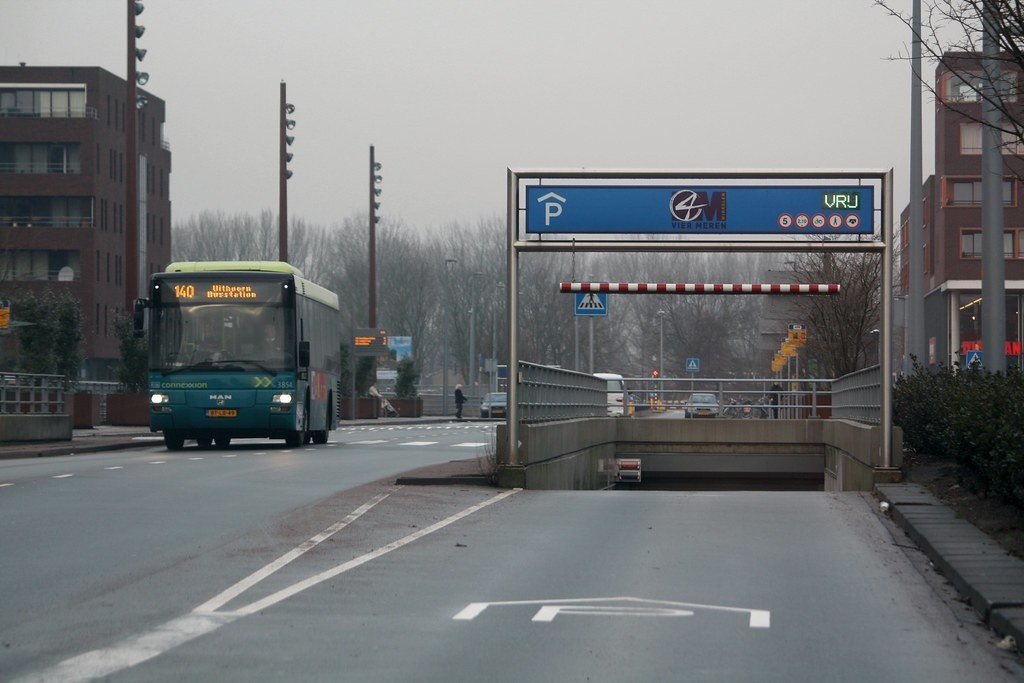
[591,373,634,418]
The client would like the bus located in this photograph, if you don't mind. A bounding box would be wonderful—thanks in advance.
[132,261,342,451]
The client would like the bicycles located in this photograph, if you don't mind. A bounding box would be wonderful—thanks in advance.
[721,397,772,419]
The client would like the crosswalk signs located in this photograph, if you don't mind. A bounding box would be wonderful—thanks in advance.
[686,358,700,373]
[574,291,608,317]
[965,350,986,371]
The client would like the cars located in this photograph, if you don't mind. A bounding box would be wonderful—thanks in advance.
[628,393,650,412]
[479,390,508,419]
[685,392,720,419]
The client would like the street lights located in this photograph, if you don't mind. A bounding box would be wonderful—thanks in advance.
[467,271,484,396]
[443,259,457,417]
[657,308,665,401]
[492,281,505,391]
[870,329,880,364]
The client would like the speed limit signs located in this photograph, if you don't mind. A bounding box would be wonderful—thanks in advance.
[777,213,794,229]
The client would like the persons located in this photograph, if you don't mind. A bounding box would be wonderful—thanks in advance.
[256,321,281,354]
[454,384,468,419]
[368,381,383,398]
[769,381,784,418]
